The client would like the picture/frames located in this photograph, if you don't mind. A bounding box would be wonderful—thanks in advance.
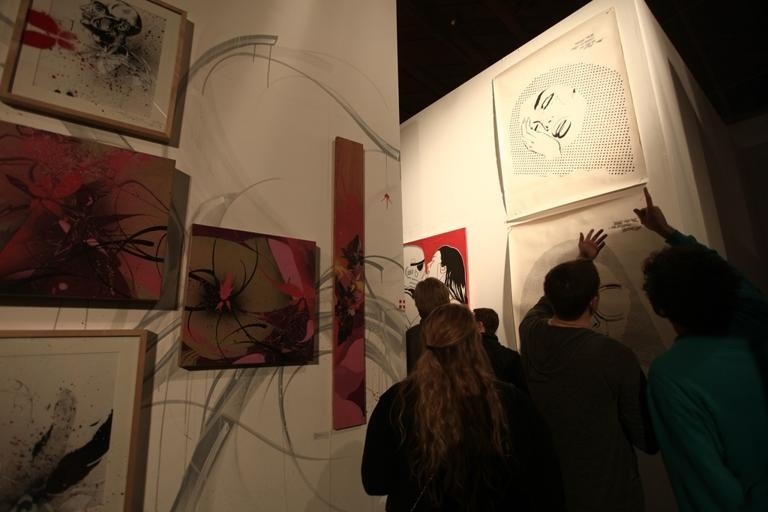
[0,328,155,512]
[0,0,189,145]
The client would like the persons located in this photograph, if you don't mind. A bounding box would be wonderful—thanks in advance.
[634,185,767,511]
[406,276,450,376]
[507,62,641,188]
[520,228,660,509]
[360,304,536,511]
[426,245,468,304]
[472,307,525,390]
